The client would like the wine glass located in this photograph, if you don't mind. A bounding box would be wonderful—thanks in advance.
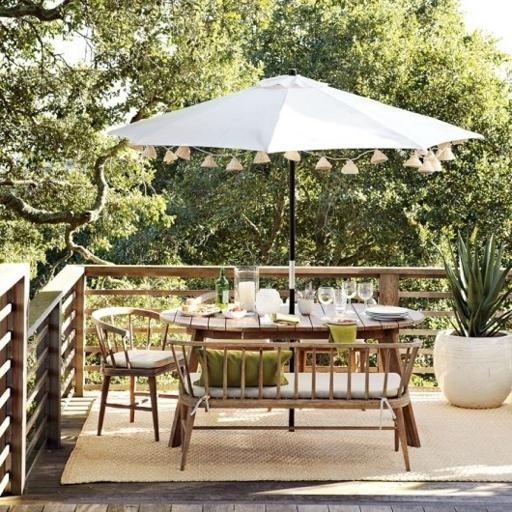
[358,283,373,316]
[343,281,356,314]
[318,286,333,321]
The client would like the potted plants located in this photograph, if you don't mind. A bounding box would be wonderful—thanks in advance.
[430,227,512,408]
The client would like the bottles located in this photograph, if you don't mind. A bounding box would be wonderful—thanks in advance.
[215,267,229,308]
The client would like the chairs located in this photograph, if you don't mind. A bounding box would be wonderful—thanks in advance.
[91,305,188,442]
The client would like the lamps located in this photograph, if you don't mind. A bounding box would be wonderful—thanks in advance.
[142,140,458,175]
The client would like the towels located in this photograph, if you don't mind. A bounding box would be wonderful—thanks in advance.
[326,323,358,353]
[272,312,299,323]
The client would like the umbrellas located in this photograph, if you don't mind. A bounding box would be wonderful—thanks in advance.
[107,71,485,256]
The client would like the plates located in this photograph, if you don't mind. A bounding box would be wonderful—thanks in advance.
[328,318,359,325]
[176,303,221,316]
[366,306,409,321]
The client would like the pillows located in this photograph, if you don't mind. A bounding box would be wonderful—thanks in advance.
[193,348,293,387]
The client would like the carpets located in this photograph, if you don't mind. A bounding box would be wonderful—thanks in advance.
[60,396,512,486]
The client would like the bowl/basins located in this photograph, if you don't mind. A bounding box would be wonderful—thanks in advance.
[221,309,247,319]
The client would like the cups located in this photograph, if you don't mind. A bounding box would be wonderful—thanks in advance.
[298,290,316,316]
[334,288,347,313]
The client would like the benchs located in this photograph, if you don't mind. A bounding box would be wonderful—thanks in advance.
[165,338,423,471]
